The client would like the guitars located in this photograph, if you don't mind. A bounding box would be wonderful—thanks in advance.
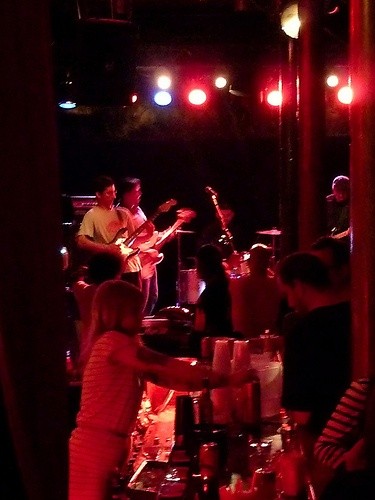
[106,199,176,261]
[126,209,196,272]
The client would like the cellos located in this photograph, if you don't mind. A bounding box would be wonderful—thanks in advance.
[206,186,275,282]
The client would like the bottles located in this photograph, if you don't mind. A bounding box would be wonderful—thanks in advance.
[166,395,199,473]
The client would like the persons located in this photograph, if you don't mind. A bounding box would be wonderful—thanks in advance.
[312,376,375,499]
[68,279,260,500]
[278,251,351,441]
[75,174,154,317]
[232,243,291,339]
[326,176,350,236]
[177,245,233,357]
[120,175,182,316]
[311,238,351,300]
[71,251,124,364]
[199,201,240,255]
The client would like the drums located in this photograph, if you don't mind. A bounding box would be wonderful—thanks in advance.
[177,268,200,304]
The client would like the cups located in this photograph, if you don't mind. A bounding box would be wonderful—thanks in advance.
[209,340,231,426]
[227,338,255,425]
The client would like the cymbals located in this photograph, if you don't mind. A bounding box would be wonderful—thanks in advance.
[159,230,195,234]
[257,229,283,235]
